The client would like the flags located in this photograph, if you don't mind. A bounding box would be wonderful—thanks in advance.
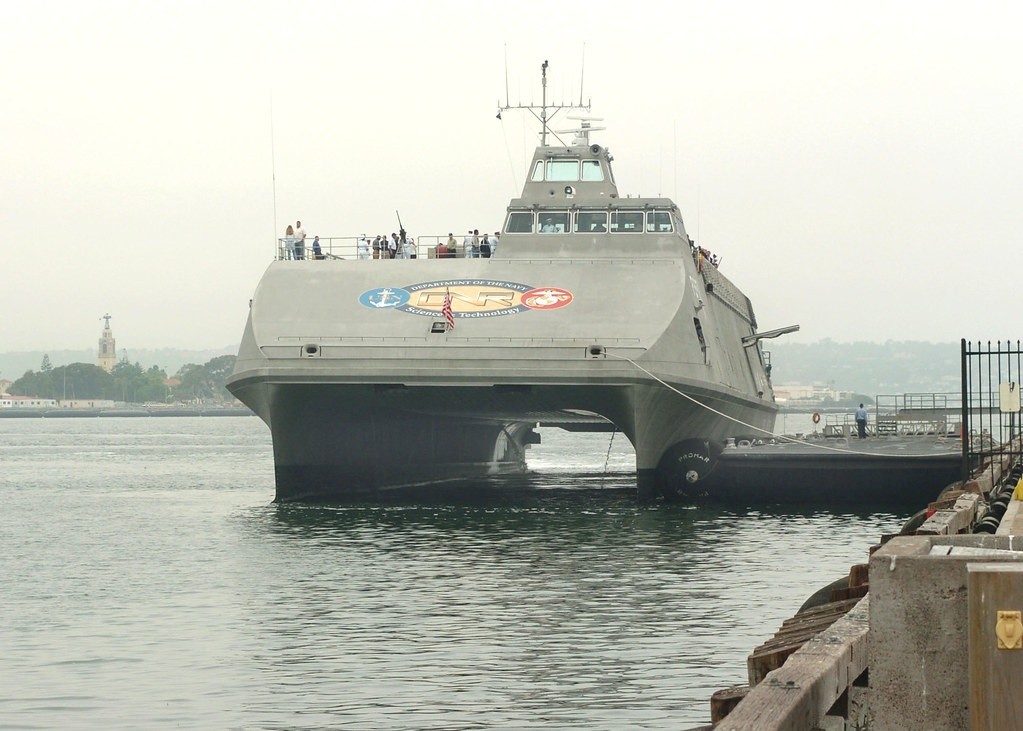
[442,290,454,330]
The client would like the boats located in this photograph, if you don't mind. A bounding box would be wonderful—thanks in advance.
[223,41,804,508]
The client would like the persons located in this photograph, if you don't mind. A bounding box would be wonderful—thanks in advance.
[357,233,417,259]
[286,220,305,260]
[313,236,324,260]
[462,229,499,258]
[446,233,457,258]
[541,218,558,233]
[592,223,606,232]
[855,403,869,439]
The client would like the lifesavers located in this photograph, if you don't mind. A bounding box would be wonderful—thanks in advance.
[698,253,703,273]
[812,412,821,424]
